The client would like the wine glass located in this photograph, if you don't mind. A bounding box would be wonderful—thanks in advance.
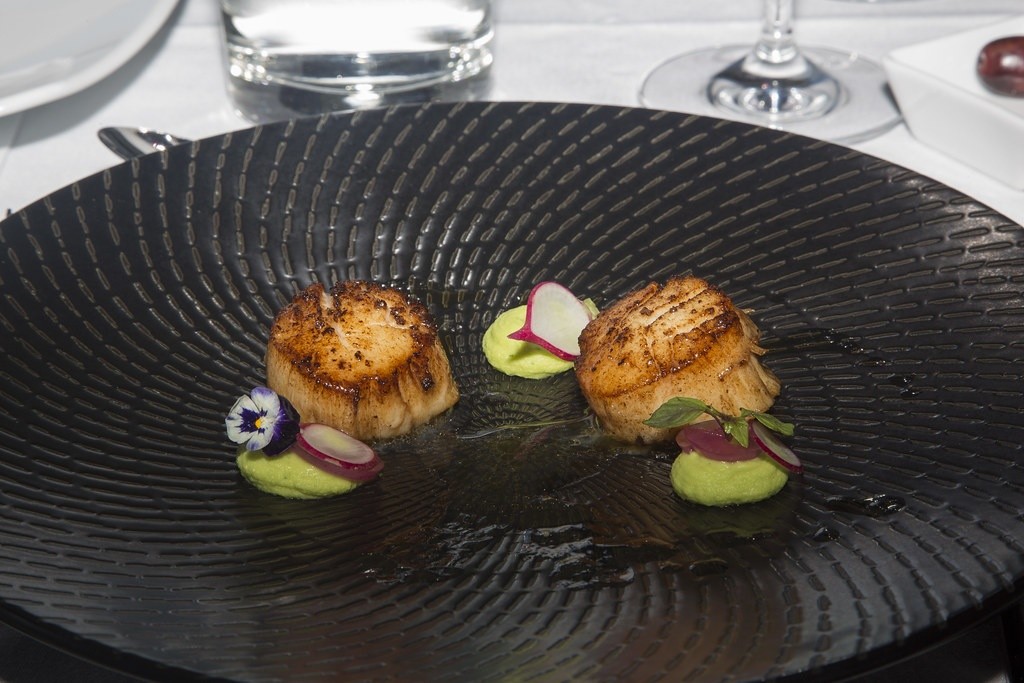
[637,0,902,144]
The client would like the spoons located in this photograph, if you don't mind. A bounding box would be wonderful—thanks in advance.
[98,126,195,162]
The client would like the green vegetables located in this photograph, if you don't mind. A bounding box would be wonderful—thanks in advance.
[642,396,795,450]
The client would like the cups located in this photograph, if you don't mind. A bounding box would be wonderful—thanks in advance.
[218,0,495,125]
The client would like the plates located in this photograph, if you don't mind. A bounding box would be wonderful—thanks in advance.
[883,14,1024,190]
[0,0,179,117]
[0,102,1023,682]
